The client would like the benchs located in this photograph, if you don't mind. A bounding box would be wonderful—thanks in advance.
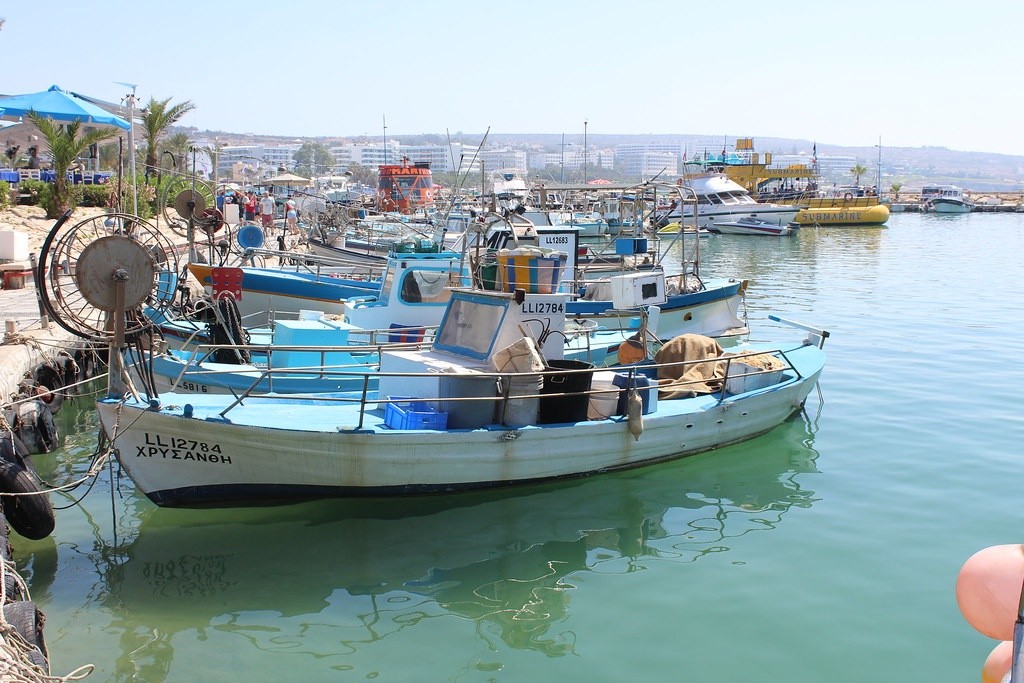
[0,260,62,289]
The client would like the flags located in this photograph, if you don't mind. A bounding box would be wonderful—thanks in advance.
[812,143,818,163]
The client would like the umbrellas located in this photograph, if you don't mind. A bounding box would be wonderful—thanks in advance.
[0,84,131,175]
[261,172,310,195]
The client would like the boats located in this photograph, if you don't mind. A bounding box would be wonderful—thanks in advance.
[932,197,972,213]
[31,122,889,506]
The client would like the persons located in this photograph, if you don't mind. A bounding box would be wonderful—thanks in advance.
[259,191,275,237]
[28,147,41,168]
[284,195,297,235]
[721,147,725,162]
[217,190,273,222]
[670,200,677,208]
[773,184,813,194]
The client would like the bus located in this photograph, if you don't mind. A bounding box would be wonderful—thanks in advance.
[922,186,963,204]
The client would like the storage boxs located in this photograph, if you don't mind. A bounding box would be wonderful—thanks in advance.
[384,396,448,431]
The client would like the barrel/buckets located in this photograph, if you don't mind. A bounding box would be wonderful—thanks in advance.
[497,372,544,427]
[587,384,620,421]
[540,359,594,423]
[428,367,504,430]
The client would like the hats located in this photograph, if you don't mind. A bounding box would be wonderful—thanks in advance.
[264,191,268,194]
